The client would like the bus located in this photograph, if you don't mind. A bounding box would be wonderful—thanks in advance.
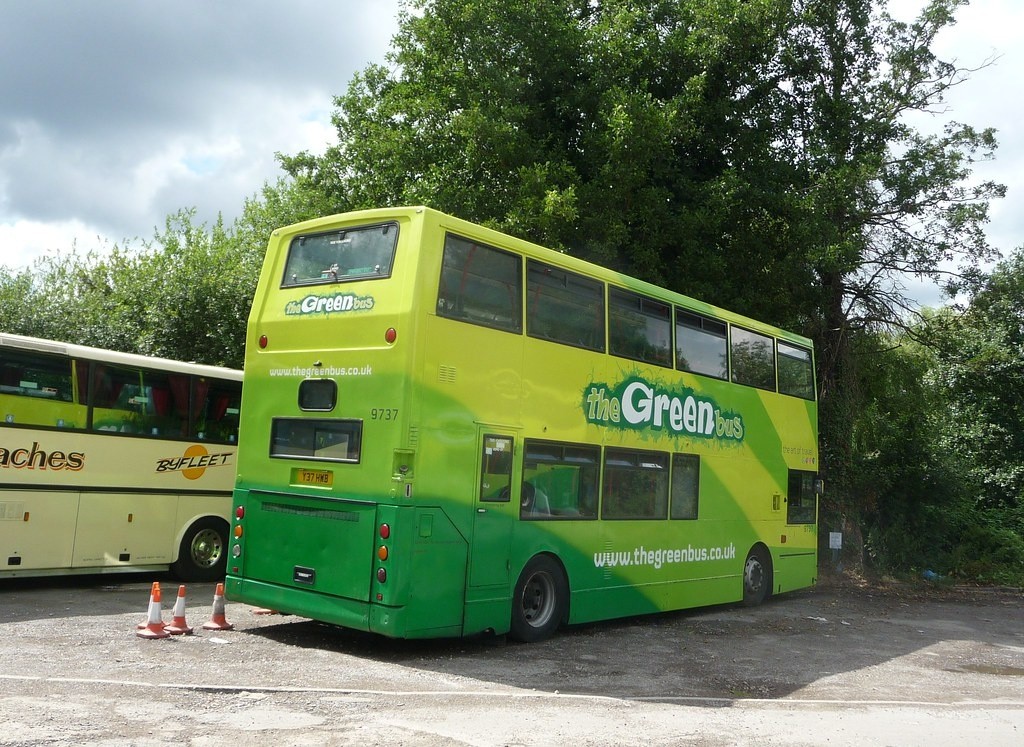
[222,204,826,644]
[0,331,244,584]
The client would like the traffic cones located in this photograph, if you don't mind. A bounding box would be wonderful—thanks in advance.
[163,584,194,636]
[135,588,171,638]
[138,582,170,629]
[201,583,234,629]
